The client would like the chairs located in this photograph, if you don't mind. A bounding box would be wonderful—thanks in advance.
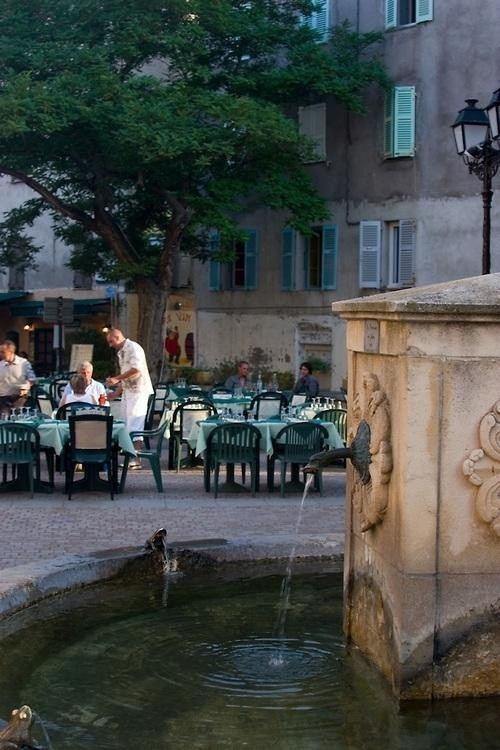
[2,423,57,499]
[23,373,178,428]
[267,424,329,497]
[312,409,347,438]
[117,416,171,493]
[205,424,263,499]
[160,370,310,444]
[64,414,116,501]
[167,402,219,473]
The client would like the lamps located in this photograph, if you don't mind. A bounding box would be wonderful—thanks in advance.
[23,318,33,336]
[101,321,112,335]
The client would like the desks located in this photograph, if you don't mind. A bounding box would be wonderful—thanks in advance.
[188,418,345,494]
[2,419,138,493]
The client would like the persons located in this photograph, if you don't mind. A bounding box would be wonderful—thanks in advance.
[293,362,319,401]
[224,360,253,388]
[59,376,97,407]
[106,329,155,470]
[64,360,110,406]
[0,340,36,420]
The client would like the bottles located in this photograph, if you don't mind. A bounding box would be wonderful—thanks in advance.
[256,373,262,395]
[99,394,106,406]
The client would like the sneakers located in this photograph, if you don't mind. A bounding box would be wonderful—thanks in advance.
[120,461,142,470]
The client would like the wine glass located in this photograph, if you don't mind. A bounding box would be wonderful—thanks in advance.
[226,407,245,422]
[311,397,336,410]
[10,406,39,421]
[281,406,308,421]
